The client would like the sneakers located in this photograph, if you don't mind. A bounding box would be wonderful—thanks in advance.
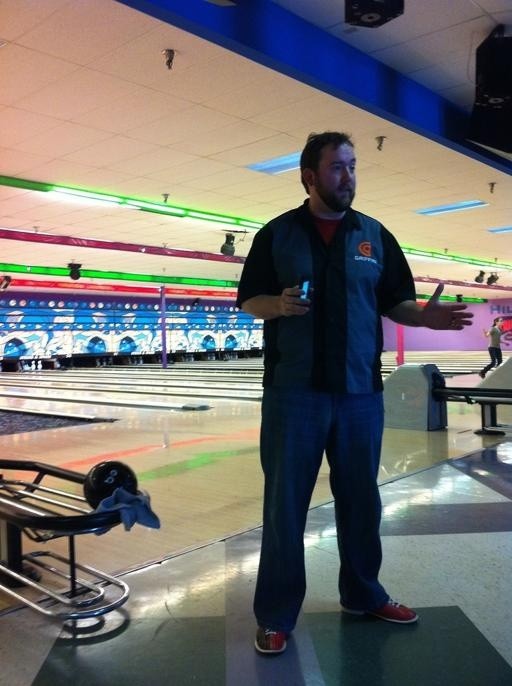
[254,625,287,655]
[339,595,419,624]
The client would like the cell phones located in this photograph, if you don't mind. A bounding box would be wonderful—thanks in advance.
[298,279,312,300]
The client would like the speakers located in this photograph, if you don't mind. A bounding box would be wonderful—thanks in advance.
[476,36,512,115]
[346,0,404,28]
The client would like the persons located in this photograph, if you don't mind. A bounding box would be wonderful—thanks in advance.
[479,317,510,379]
[237,132,474,654]
